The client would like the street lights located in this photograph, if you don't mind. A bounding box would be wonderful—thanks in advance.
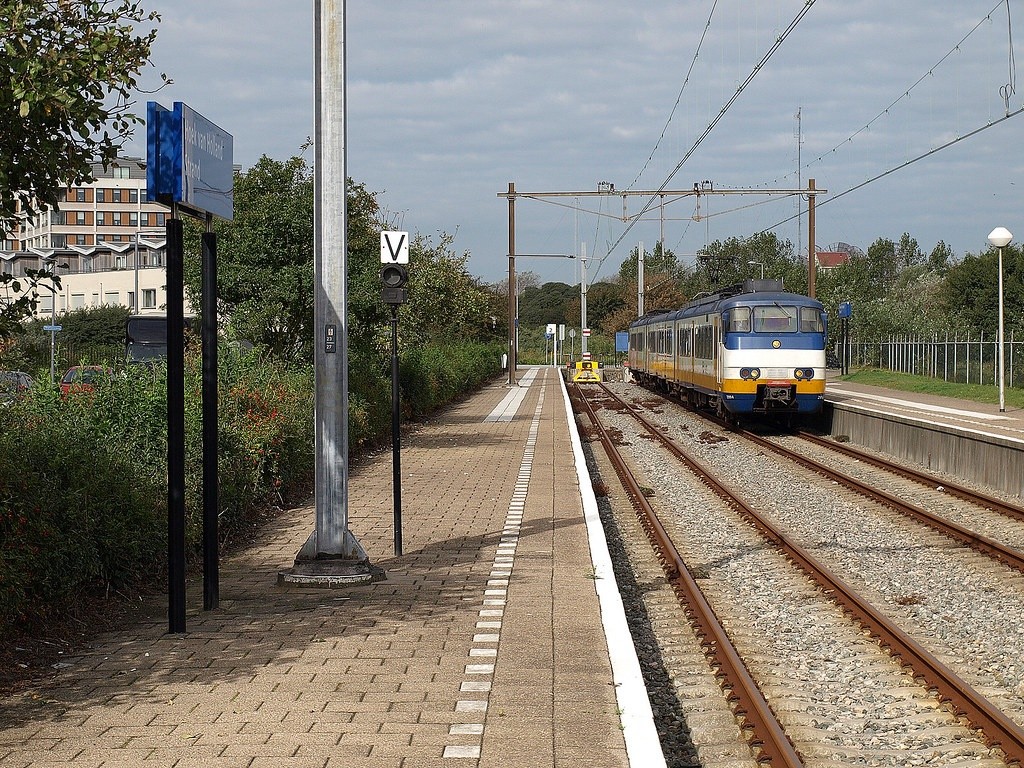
[988,227,1015,412]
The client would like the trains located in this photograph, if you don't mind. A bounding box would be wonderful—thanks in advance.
[626,278,828,430]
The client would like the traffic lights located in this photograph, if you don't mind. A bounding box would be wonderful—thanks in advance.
[381,263,405,303]
